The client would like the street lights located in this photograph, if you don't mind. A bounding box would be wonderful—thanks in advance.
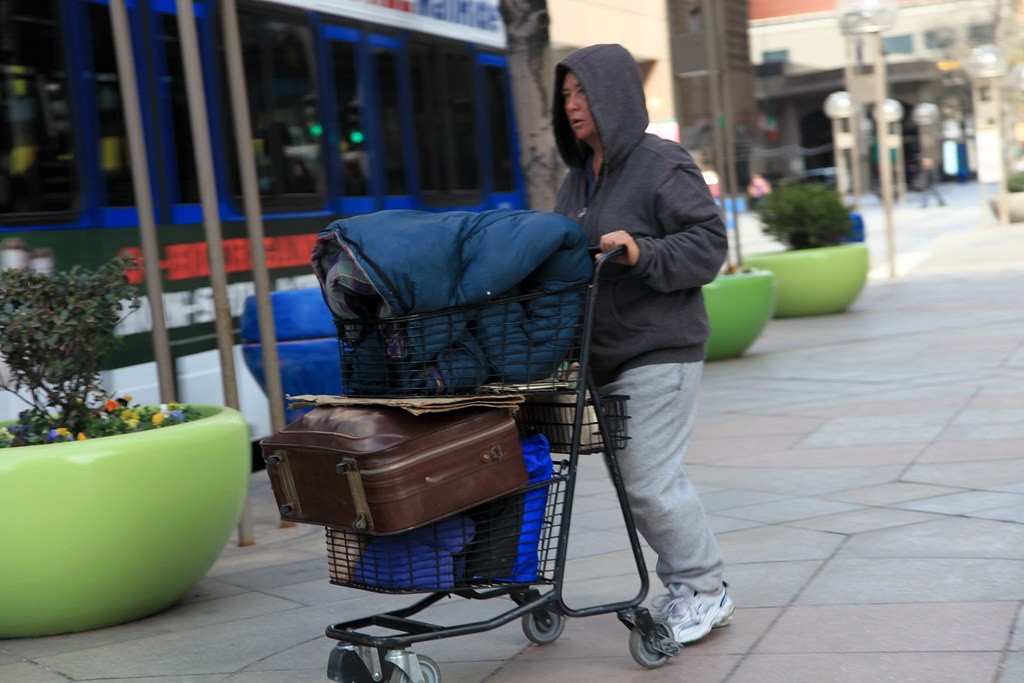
[912,101,939,188]
[873,99,904,203]
[838,0,900,280]
[826,92,857,205]
[967,47,1010,225]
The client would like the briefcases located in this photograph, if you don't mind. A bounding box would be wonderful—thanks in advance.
[259,407,529,533]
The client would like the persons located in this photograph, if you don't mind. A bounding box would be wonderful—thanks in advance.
[551,43,736,643]
[920,156,945,208]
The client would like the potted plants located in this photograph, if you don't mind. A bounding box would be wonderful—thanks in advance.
[988,169,1024,222]
[1,248,253,643]
[743,177,871,317]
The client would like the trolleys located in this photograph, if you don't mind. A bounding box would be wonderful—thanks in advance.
[323,243,683,683]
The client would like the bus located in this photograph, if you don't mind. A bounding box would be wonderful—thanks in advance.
[0,0,523,447]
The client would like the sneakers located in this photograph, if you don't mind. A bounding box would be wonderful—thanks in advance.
[647,583,735,644]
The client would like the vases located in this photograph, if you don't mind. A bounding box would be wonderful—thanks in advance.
[701,262,775,363]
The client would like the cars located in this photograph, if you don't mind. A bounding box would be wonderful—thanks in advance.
[780,167,836,192]
